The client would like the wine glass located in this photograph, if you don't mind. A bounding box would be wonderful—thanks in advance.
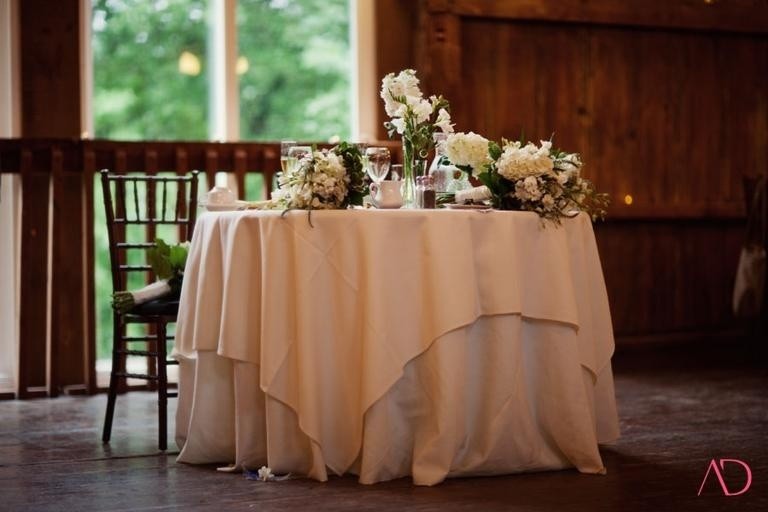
[280,140,472,211]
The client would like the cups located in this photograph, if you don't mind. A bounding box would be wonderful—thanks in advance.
[194,184,236,204]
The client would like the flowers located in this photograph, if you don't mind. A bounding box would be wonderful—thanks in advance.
[439,132,611,229]
[380,64,452,194]
[254,139,368,230]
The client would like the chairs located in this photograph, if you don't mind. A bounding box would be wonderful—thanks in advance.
[97,167,202,451]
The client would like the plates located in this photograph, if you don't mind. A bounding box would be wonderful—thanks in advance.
[205,205,236,211]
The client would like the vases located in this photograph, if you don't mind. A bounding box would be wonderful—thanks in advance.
[415,157,429,208]
[396,132,415,210]
